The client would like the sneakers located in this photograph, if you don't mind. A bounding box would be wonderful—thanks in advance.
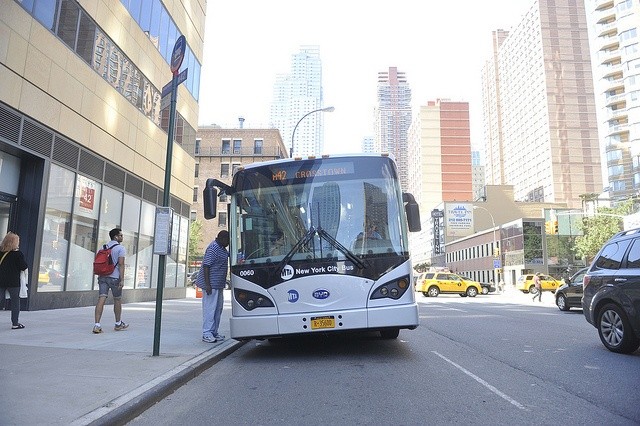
[92,325,102,333]
[12,323,25,329]
[202,333,217,343]
[114,322,129,330]
[214,334,225,341]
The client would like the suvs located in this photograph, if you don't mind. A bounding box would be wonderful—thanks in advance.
[38,265,49,285]
[190,271,231,290]
[516,274,565,293]
[581,226,640,352]
[416,271,482,297]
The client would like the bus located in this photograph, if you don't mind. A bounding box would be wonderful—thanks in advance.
[203,153,421,345]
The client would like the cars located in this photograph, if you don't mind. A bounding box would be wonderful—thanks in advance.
[49,268,76,284]
[459,276,496,297]
[555,266,589,310]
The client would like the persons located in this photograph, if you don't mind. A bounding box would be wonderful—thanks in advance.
[196,230,229,343]
[356,215,383,240]
[266,230,285,257]
[93,229,130,333]
[0,231,29,329]
[490,279,494,287]
[498,279,504,291]
[532,272,543,302]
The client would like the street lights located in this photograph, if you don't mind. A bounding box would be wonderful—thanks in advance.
[290,107,335,157]
[473,205,500,292]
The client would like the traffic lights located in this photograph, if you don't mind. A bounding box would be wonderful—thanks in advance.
[494,248,499,256]
[552,220,559,235]
[544,220,551,234]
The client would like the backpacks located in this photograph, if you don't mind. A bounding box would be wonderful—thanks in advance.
[93,244,120,275]
